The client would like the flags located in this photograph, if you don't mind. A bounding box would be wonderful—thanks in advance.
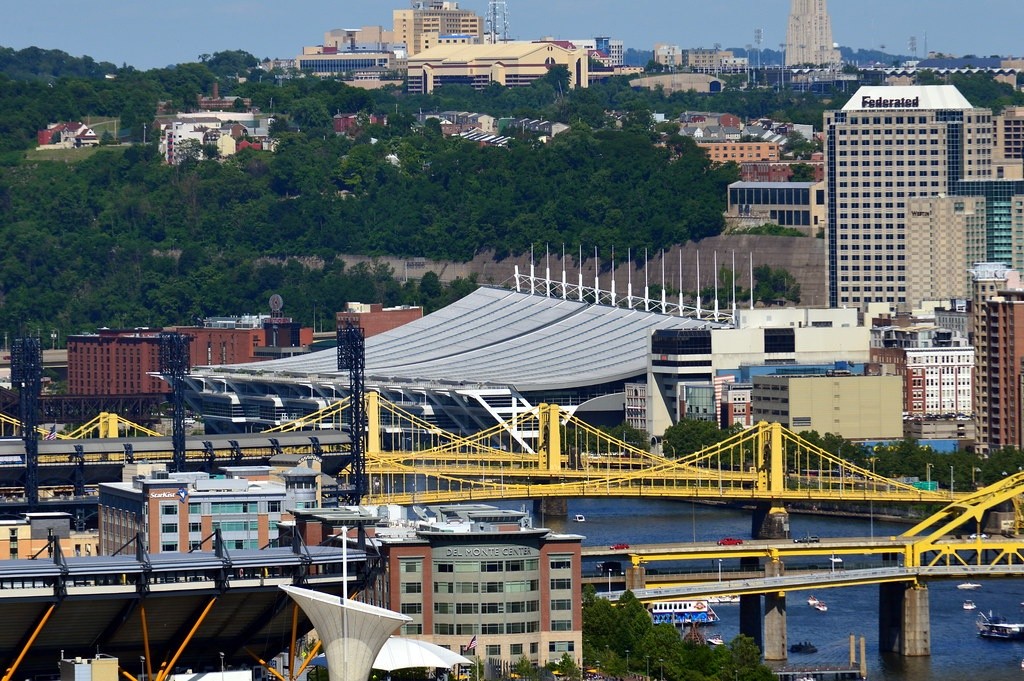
[463,635,477,654]
[43,425,56,440]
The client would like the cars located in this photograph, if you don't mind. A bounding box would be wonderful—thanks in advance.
[793,536,821,544]
[968,533,988,539]
[610,543,629,551]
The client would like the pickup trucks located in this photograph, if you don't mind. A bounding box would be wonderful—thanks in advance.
[717,537,743,546]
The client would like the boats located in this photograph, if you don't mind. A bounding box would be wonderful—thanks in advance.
[962,599,976,610]
[807,595,828,612]
[791,641,818,654]
[957,583,982,591]
[975,609,1024,642]
[706,635,725,646]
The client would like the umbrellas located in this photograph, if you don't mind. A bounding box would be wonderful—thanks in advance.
[306,634,474,681]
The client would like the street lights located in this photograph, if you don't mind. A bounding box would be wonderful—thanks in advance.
[949,465,954,499]
[734,670,738,681]
[794,450,802,473]
[929,463,935,491]
[608,568,612,593]
[584,639,666,681]
[718,558,723,582]
[742,447,751,463]
[870,456,879,492]
[972,467,982,483]
[702,443,709,467]
[650,435,676,460]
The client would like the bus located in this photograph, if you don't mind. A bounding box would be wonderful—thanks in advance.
[802,469,840,477]
